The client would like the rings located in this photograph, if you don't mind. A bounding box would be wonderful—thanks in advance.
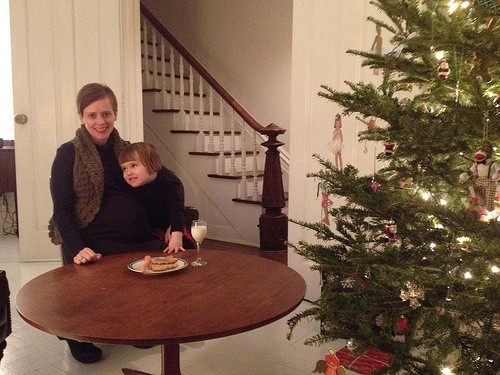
[79,257,83,260]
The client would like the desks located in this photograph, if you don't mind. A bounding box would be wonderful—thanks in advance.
[14,249,307,375]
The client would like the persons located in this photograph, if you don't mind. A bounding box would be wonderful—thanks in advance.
[48,83,196,363]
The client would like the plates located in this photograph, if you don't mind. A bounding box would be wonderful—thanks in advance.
[126,256,188,275]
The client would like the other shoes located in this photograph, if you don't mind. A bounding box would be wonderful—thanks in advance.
[57,336,103,364]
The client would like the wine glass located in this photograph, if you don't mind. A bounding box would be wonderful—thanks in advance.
[190,219,208,268]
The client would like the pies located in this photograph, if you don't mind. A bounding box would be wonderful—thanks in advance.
[150,256,178,271]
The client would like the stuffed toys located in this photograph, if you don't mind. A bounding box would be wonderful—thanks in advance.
[459,148,500,211]
[438,59,451,80]
